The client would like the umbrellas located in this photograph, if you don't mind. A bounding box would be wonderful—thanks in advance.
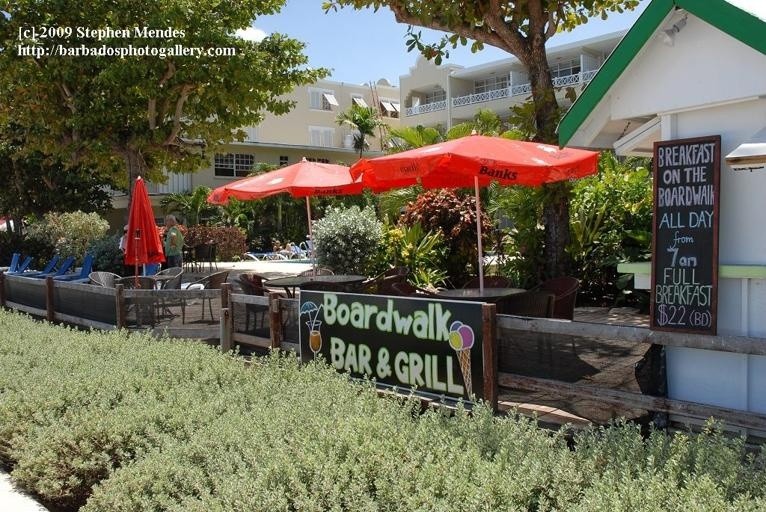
[299,301,318,328]
[348,129,600,289]
[207,157,365,276]
[124,176,167,288]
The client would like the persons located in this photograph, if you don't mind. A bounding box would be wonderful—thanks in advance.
[162,213,184,289]
[117,224,134,275]
[273,239,295,254]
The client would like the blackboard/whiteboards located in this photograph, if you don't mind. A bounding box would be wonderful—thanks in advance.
[650,134,721,335]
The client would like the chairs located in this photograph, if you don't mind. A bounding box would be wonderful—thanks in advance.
[6,241,581,373]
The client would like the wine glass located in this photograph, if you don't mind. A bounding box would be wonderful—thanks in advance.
[305,320,323,361]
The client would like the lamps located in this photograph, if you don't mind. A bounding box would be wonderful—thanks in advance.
[658,15,688,50]
